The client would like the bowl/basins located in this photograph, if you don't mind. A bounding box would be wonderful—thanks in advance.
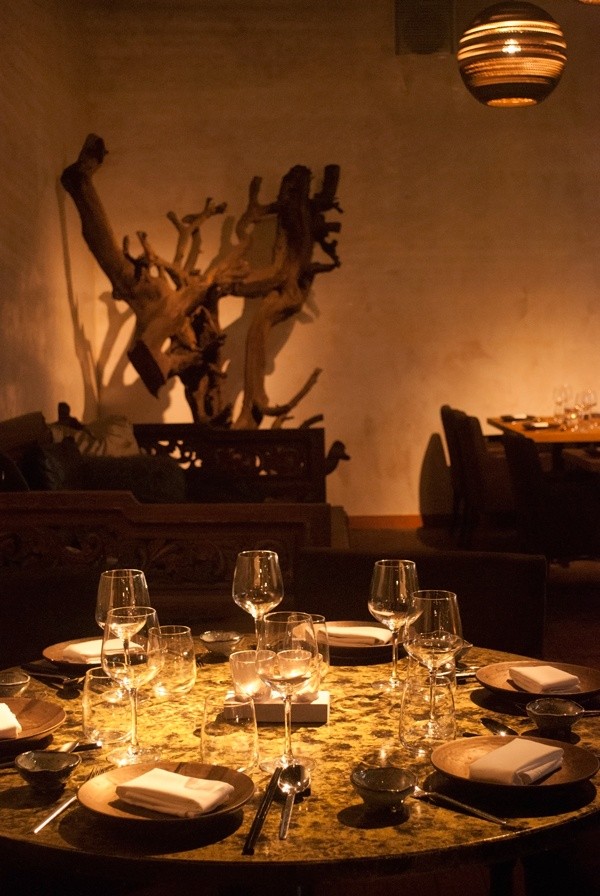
[78,762,255,828]
[42,635,158,671]
[349,765,418,812]
[430,736,600,806]
[199,629,244,657]
[15,750,80,793]
[293,622,420,656]
[476,660,600,705]
[526,697,586,738]
[0,671,31,698]
[424,641,475,668]
[0,698,67,747]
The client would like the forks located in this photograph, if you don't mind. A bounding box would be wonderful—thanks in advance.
[29,767,104,836]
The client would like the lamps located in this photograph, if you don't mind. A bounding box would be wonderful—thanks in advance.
[456,0,569,109]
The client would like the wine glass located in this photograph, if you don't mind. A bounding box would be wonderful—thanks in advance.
[549,386,597,432]
[96,548,465,766]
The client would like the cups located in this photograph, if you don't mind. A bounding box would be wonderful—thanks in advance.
[276,649,322,703]
[229,649,276,702]
[283,614,332,684]
[397,675,462,758]
[202,690,259,766]
[149,624,197,699]
[81,664,134,746]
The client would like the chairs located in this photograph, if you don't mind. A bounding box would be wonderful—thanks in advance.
[439,405,526,551]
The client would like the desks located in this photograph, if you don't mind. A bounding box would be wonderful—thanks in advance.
[487,418,600,554]
[0,630,600,896]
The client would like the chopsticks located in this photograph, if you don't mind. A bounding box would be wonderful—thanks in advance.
[243,767,283,852]
[0,743,102,764]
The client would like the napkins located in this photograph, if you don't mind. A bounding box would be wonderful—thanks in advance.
[320,625,393,645]
[508,665,582,694]
[117,767,234,819]
[469,739,563,785]
[63,636,145,663]
[0,702,22,739]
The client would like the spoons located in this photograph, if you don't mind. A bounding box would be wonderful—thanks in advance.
[279,764,312,838]
[25,671,85,687]
